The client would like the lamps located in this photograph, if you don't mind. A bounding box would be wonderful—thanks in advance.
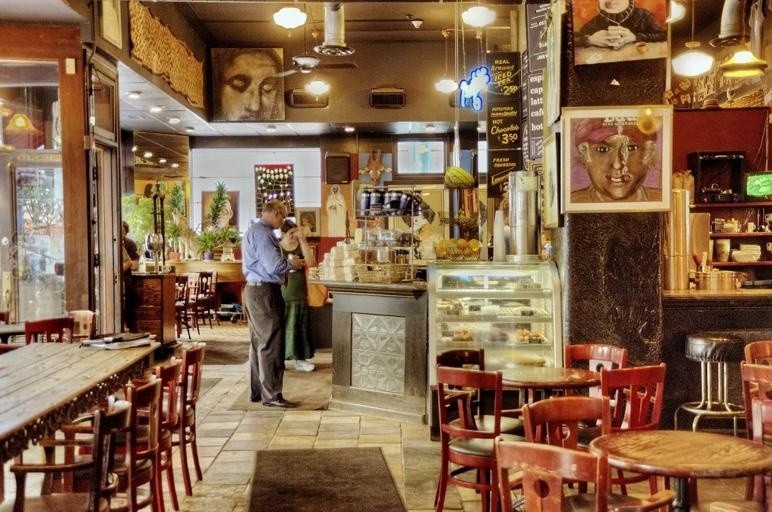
[460,0,498,34]
[718,0,768,80]
[269,0,310,31]
[668,0,720,80]
[1,83,43,135]
[433,24,460,95]
[304,29,332,97]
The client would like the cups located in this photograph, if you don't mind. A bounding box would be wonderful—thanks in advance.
[711,217,743,233]
[357,228,411,265]
[708,240,714,261]
[746,222,756,233]
[493,208,506,263]
[165,260,176,273]
[308,267,319,280]
[716,239,731,262]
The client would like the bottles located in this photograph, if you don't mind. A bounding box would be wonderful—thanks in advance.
[139,252,147,274]
[542,238,553,262]
[360,190,422,216]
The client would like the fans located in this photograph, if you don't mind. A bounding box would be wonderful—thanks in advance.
[266,0,360,80]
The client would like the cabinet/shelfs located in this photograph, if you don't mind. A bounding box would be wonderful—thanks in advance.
[428,259,562,425]
[690,197,772,271]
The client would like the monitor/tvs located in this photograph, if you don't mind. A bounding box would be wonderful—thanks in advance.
[743,171,772,202]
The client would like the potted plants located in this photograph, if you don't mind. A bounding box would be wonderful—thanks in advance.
[192,227,224,261]
[217,227,245,262]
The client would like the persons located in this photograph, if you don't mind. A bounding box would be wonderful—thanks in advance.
[326,184,348,238]
[571,118,663,203]
[574,0,666,50]
[241,198,300,407]
[278,220,316,372]
[120,231,135,334]
[214,48,283,122]
[122,222,139,271]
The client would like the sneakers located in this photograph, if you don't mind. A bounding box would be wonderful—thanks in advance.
[294,362,315,372]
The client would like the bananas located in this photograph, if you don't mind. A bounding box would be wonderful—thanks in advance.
[445,166,475,187]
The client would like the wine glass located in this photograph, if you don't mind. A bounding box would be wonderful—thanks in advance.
[146,233,165,275]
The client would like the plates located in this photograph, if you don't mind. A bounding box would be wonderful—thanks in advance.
[732,244,760,262]
[318,245,355,282]
[698,189,736,202]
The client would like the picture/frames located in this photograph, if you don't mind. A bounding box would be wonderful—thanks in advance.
[558,101,677,215]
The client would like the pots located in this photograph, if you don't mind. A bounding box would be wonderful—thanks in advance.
[695,266,737,290]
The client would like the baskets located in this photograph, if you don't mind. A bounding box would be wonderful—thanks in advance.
[355,263,406,285]
[438,211,478,225]
[443,178,476,191]
[434,245,480,261]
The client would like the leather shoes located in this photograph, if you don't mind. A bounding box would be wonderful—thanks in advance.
[265,399,299,408]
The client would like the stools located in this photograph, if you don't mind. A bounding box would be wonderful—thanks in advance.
[672,334,746,439]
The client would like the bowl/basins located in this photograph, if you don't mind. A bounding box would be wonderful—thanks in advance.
[445,244,478,261]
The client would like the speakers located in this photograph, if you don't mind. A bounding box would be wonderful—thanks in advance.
[325,152,350,184]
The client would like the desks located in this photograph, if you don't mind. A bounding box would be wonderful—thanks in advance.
[131,272,175,343]
[588,428,772,510]
[1,342,162,466]
[495,366,601,448]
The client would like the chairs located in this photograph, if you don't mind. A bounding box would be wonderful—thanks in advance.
[492,434,612,511]
[135,340,207,498]
[744,341,769,367]
[562,343,628,399]
[562,362,667,498]
[51,375,166,510]
[169,265,218,339]
[77,357,183,511]
[520,394,634,511]
[740,360,771,500]
[1,396,133,510]
[1,309,95,357]
[750,397,770,504]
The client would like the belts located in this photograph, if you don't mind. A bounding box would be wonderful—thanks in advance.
[246,279,277,287]
